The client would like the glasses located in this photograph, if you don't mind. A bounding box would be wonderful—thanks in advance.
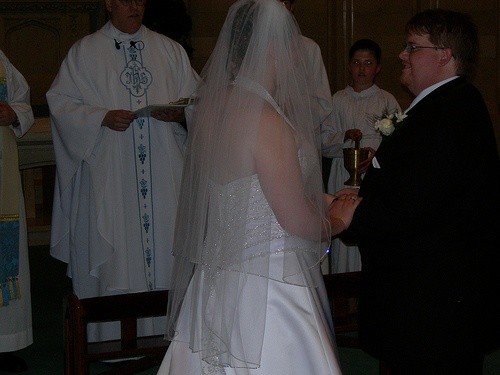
[400,41,445,52]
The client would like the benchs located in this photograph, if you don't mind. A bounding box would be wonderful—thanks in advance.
[63,271,387,375]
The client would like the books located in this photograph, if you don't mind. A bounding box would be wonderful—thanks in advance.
[134,103,188,117]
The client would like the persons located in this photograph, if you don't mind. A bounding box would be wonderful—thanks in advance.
[157,0,363,375]
[46,0,205,363]
[0,48,35,375]
[336,8,500,375]
[281,0,333,126]
[320,38,403,290]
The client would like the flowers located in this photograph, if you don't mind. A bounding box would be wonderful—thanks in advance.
[369,106,408,136]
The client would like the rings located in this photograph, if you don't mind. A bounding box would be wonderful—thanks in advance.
[350,197,356,201]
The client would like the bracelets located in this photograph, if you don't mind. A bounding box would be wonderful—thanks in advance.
[328,197,339,208]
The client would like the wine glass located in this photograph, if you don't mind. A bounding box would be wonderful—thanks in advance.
[343,148,370,186]
